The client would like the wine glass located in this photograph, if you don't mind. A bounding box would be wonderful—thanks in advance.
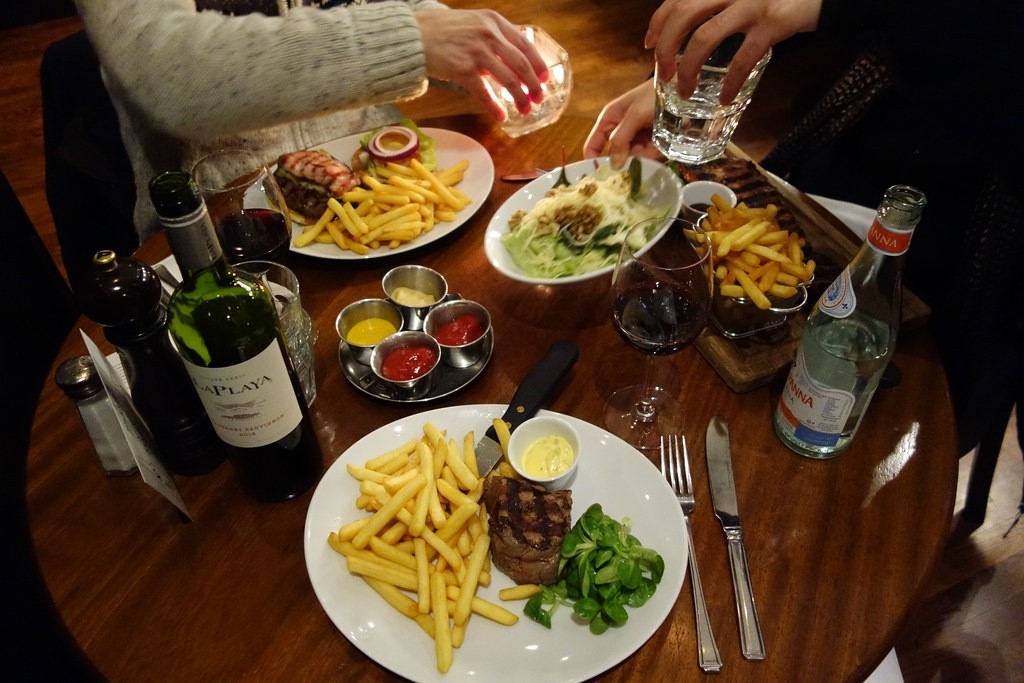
[604,217,712,449]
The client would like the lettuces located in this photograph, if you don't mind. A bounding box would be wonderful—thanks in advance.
[359,116,439,171]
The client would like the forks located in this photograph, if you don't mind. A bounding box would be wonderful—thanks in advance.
[659,435,723,671]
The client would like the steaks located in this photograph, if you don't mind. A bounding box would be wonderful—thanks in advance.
[481,475,573,587]
[676,157,823,294]
[264,151,361,219]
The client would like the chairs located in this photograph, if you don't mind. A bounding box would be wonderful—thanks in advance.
[0,173,111,683]
[945,319,1024,553]
[39,29,137,302]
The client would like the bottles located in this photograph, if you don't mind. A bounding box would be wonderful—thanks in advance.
[55,170,321,506]
[772,184,927,459]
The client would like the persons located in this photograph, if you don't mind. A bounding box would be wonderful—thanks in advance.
[74,1,548,259]
[582,0,1023,424]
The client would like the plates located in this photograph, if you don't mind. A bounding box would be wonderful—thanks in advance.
[302,403,689,683]
[484,154,682,285]
[337,295,494,401]
[242,126,495,259]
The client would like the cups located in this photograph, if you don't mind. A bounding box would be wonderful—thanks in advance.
[481,24,573,136]
[190,148,291,264]
[235,259,317,407]
[651,47,772,166]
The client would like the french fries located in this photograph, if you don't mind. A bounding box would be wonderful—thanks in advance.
[328,413,543,673]
[682,194,817,310]
[293,158,473,254]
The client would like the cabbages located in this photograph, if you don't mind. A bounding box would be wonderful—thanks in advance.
[503,158,674,281]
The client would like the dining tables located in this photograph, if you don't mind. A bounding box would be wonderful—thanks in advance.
[25,113,961,683]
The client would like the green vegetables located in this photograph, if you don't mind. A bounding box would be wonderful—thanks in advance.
[523,503,665,634]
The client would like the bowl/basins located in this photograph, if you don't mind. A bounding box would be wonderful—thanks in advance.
[335,264,492,399]
[508,420,581,490]
[682,181,738,224]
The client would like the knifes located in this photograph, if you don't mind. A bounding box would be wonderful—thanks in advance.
[475,339,581,477]
[706,413,766,660]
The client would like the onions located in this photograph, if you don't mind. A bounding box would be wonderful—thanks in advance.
[365,126,421,160]
